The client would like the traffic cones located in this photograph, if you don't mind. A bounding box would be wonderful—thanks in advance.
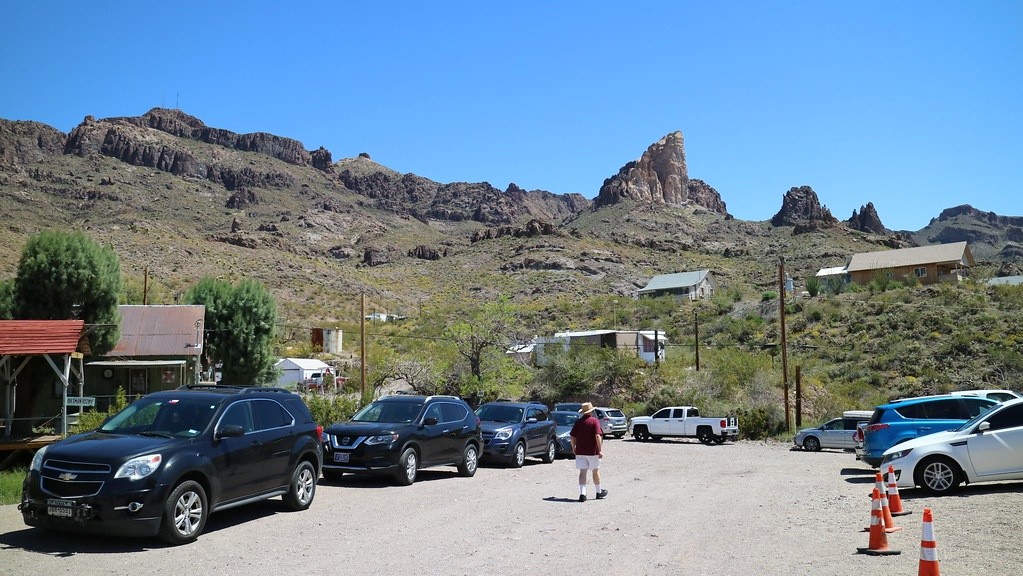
[857,488,902,556]
[887,466,912,516]
[863,472,903,534]
[917,507,940,576]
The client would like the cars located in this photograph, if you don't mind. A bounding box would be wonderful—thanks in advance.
[881,398,1023,495]
[549,411,583,458]
[592,407,625,440]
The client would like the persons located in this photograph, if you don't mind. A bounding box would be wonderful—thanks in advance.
[569,402,608,501]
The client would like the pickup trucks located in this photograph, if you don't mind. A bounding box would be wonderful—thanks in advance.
[628,406,739,445]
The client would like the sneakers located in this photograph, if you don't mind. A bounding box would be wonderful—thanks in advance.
[596,489,608,499]
[579,494,587,502]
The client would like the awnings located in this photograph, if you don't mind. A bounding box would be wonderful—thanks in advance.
[644,335,667,340]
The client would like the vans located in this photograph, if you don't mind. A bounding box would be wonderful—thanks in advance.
[891,389,1023,416]
[794,410,875,452]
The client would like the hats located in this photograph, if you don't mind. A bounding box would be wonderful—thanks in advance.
[578,402,597,414]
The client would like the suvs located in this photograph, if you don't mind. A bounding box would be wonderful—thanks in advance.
[17,385,324,547]
[321,395,485,486]
[553,402,602,422]
[859,395,1001,469]
[472,398,557,468]
